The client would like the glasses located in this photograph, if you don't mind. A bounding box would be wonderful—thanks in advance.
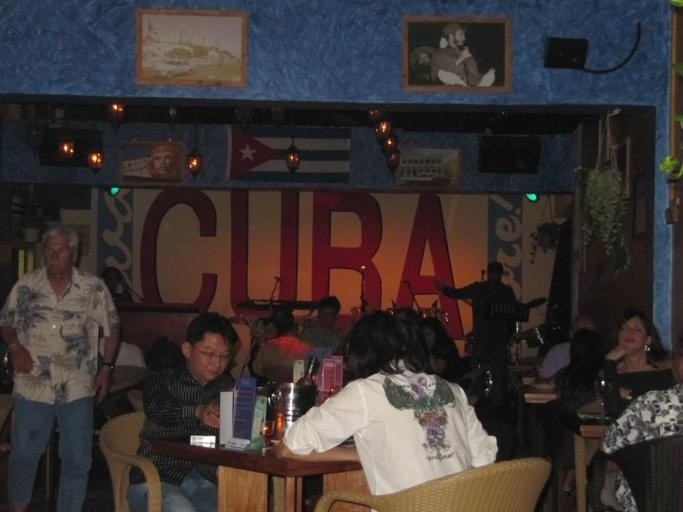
[190,341,234,361]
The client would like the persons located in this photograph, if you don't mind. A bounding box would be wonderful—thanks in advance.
[599,336,682,511]
[599,307,675,418]
[140,143,183,185]
[431,258,548,381]
[428,22,496,89]
[0,224,124,512]
[398,308,521,460]
[535,312,619,493]
[274,310,499,511]
[127,311,238,511]
[247,295,348,384]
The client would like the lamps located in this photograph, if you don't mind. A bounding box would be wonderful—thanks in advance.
[285,132,299,175]
[84,141,103,181]
[108,100,126,132]
[365,103,398,166]
[185,126,201,186]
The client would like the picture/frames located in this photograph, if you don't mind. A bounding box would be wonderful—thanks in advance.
[393,138,463,191]
[114,138,192,190]
[399,11,510,94]
[132,5,249,91]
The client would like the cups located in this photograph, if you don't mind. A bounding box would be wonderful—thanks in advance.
[260,419,274,436]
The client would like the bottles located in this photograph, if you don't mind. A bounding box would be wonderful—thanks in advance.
[296,355,319,385]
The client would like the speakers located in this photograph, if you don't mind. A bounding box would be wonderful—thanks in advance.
[478,136,540,174]
[39,127,103,167]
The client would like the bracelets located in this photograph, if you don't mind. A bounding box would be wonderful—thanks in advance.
[8,342,17,350]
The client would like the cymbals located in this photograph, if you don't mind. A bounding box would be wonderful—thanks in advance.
[525,298,547,309]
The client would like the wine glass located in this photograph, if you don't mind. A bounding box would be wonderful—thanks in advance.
[594,378,613,419]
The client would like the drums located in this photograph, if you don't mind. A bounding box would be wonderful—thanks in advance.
[463,333,477,357]
[420,309,448,325]
[524,321,560,347]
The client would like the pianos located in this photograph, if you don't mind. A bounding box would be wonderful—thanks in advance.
[238,300,320,311]
[113,301,202,356]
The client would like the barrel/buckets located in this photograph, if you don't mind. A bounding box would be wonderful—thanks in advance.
[268,382,317,436]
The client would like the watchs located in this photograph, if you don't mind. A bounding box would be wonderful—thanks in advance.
[101,359,117,372]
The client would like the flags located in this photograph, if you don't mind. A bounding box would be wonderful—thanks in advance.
[225,120,352,184]
